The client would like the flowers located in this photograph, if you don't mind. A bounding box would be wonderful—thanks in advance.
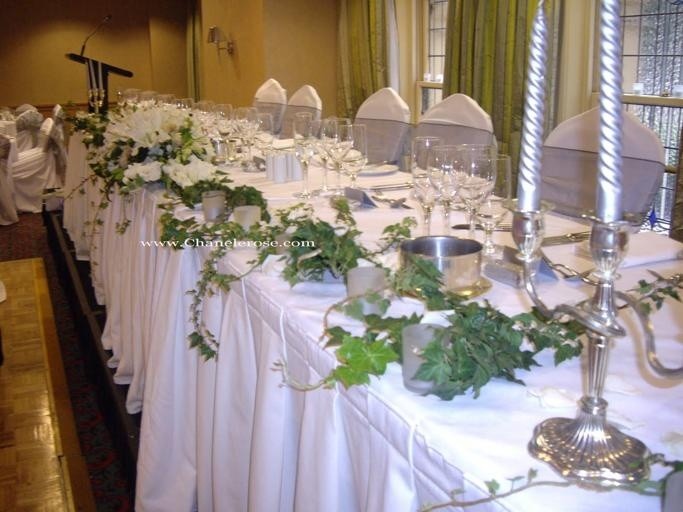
[96,98,225,184]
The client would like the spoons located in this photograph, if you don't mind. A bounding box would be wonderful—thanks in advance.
[541,250,595,281]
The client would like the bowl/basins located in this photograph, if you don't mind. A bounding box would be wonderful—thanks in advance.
[399,234,486,292]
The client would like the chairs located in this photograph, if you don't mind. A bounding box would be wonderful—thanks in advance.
[530,108,664,225]
[242,79,324,135]
[1,103,65,225]
[343,85,496,184]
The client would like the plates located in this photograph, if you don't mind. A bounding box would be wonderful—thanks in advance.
[356,162,400,178]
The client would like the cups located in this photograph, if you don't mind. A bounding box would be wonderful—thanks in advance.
[200,190,226,223]
[345,266,386,319]
[632,82,644,96]
[232,205,262,242]
[423,72,430,81]
[397,323,447,399]
[435,74,442,82]
[672,84,683,98]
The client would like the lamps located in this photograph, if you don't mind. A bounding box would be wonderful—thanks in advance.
[208,26,235,55]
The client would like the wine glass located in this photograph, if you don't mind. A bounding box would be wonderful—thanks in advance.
[335,123,366,196]
[408,135,442,236]
[425,143,468,238]
[121,88,276,173]
[321,117,354,196]
[290,118,322,201]
[468,153,511,257]
[306,118,338,198]
[450,143,497,241]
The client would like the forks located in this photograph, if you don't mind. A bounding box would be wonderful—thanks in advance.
[538,227,591,248]
[451,220,513,233]
[543,252,598,286]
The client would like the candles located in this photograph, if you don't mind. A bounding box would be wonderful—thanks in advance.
[514,2,552,215]
[597,0,622,231]
